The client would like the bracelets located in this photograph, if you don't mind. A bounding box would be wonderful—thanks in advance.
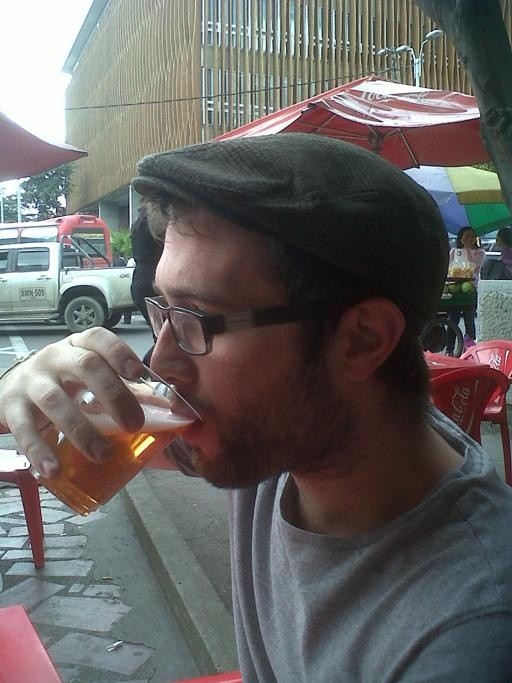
[0,347,39,380]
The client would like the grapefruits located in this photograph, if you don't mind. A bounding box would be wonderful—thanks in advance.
[442,281,475,294]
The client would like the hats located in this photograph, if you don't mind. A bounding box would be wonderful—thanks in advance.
[130,134,449,326]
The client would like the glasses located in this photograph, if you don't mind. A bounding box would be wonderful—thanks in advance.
[143,293,338,357]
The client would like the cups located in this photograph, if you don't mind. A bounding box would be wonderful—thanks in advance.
[28,359,203,518]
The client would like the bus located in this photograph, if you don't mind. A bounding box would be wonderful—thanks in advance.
[0,214,113,270]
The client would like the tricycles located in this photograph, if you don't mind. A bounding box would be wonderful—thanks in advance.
[420,274,478,359]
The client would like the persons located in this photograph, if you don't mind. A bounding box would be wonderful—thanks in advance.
[494,225,511,278]
[0,134,510,681]
[446,223,486,359]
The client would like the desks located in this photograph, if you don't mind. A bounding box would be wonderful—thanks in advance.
[0,604,65,683]
[424,352,490,378]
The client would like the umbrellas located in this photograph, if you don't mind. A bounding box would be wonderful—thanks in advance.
[397,160,511,235]
[196,72,491,170]
[0,107,86,182]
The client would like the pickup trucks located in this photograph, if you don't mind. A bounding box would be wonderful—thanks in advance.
[0,240,134,332]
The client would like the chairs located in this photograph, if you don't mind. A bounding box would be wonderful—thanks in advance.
[0,425,45,570]
[429,365,510,444]
[460,339,512,488]
